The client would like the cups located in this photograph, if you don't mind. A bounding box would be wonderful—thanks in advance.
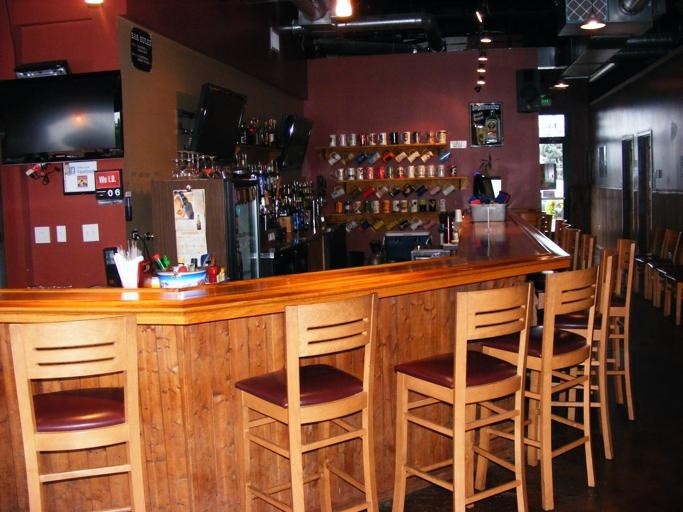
[474,124,488,145]
[334,199,446,212]
[345,218,437,231]
[328,165,455,200]
[453,208,463,223]
[327,130,451,165]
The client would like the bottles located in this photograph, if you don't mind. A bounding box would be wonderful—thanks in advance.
[443,214,452,244]
[236,153,279,175]
[150,250,226,285]
[484,106,500,144]
[263,177,312,232]
[238,116,279,147]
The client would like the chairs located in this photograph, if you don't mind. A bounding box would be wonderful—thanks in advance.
[635,228,680,326]
[236,287,382,512]
[391,280,538,511]
[7,314,148,510]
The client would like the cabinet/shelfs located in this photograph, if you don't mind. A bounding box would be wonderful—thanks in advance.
[322,141,469,223]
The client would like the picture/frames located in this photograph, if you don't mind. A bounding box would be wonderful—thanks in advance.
[469,101,503,149]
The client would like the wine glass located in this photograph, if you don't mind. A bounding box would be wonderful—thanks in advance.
[171,149,217,179]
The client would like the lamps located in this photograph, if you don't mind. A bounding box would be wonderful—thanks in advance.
[472,0,494,94]
[580,2,606,30]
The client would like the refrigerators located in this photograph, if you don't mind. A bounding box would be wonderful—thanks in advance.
[148,176,261,282]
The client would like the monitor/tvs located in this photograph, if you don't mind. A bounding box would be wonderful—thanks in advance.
[515,67,584,114]
[279,113,314,173]
[0,69,124,164]
[189,82,248,164]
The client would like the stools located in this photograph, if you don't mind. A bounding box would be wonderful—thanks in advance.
[534,211,596,312]
[591,237,636,421]
[473,264,602,510]
[539,248,615,460]
